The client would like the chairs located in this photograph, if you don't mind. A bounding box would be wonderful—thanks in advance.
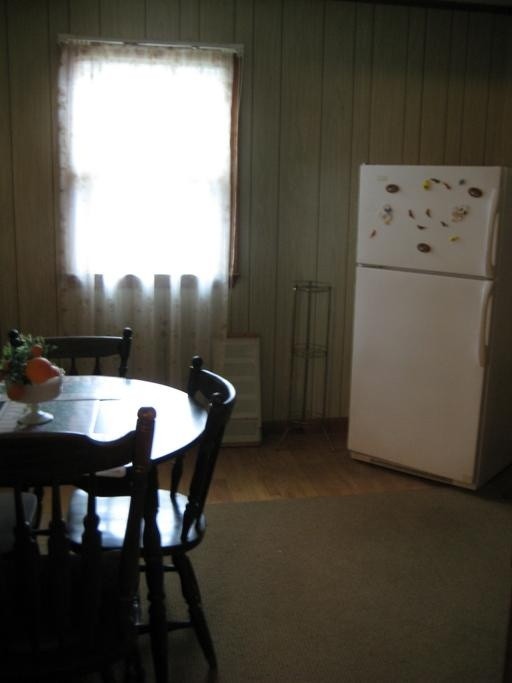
[0,404,156,683]
[0,486,39,554]
[67,353,237,670]
[7,325,135,547]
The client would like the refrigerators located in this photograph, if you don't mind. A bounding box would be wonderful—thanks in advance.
[345,162,512,494]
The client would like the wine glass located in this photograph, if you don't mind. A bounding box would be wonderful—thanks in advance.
[3,369,65,425]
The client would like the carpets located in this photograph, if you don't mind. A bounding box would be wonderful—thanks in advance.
[147,482,512,682]
